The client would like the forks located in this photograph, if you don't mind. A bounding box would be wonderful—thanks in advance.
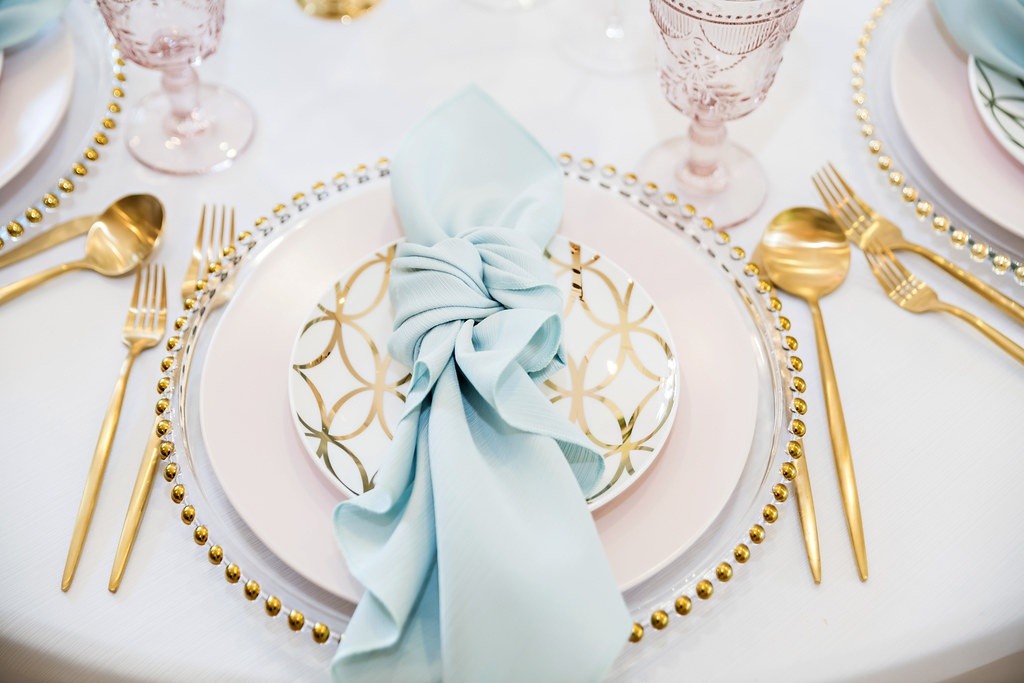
[861,234,1024,365]
[813,161,1024,324]
[61,261,168,593]
[108,204,237,593]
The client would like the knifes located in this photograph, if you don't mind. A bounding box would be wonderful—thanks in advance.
[0,215,99,270]
[788,437,820,584]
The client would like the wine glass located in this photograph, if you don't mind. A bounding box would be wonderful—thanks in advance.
[632,0,805,229]
[96,0,257,173]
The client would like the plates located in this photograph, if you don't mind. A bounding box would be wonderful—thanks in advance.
[0,0,122,251]
[156,155,807,654]
[853,0,1024,285]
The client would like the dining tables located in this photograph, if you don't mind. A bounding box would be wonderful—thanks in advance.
[0,0,1024,683]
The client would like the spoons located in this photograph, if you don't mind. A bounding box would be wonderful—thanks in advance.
[761,208,869,584]
[0,195,166,302]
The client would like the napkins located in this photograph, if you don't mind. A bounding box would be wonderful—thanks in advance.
[327,89,630,681]
